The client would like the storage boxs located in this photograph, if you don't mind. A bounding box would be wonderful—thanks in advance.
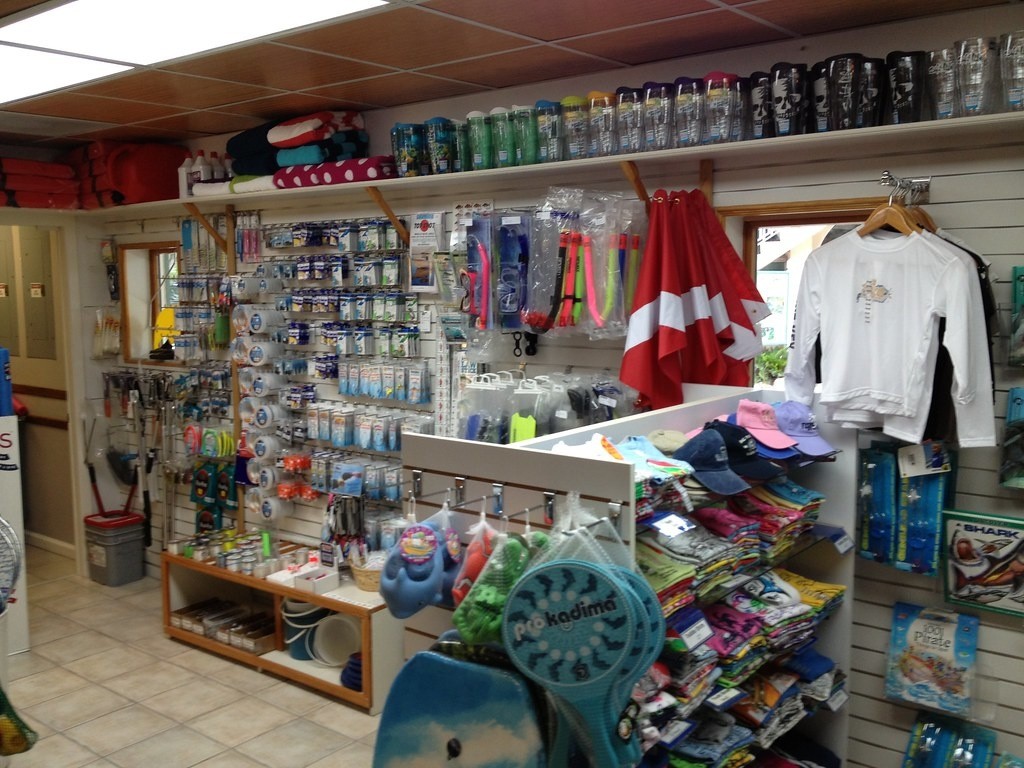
[203,606,252,637]
[231,616,273,648]
[294,568,340,594]
[182,601,233,630]
[193,605,232,636]
[171,594,223,627]
[216,614,274,644]
[242,625,276,654]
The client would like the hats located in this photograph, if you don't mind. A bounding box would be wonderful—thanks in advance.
[648,399,837,496]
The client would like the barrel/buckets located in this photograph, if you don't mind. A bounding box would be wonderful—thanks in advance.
[280,599,361,667]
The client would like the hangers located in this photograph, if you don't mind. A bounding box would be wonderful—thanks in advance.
[808,173,970,259]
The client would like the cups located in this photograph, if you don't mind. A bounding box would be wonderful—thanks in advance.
[389,31,1024,178]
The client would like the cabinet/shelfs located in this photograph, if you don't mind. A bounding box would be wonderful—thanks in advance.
[619,432,859,768]
[153,539,405,720]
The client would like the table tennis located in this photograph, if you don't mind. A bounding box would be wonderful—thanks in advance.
[500,557,667,768]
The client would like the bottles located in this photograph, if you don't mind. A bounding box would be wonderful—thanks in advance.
[176,148,233,199]
